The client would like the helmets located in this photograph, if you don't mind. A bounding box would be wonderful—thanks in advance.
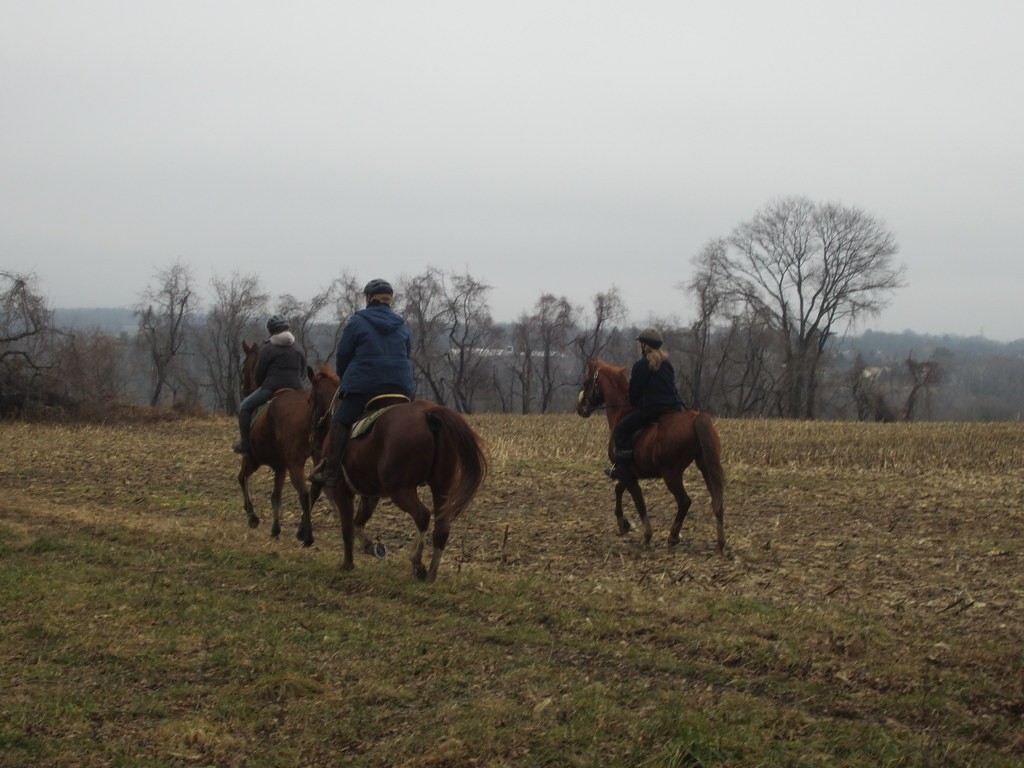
[267,315,289,334]
[362,278,393,296]
[636,327,662,348]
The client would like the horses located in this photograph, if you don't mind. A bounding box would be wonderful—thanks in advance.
[574,355,729,559]
[293,362,493,585]
[234,338,324,550]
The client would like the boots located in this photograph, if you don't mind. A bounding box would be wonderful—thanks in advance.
[313,420,349,486]
[604,450,632,480]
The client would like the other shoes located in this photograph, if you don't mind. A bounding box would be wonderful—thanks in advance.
[233,441,248,452]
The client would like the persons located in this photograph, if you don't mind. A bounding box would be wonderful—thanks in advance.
[603,327,681,479]
[309,278,415,486]
[232,314,307,453]
